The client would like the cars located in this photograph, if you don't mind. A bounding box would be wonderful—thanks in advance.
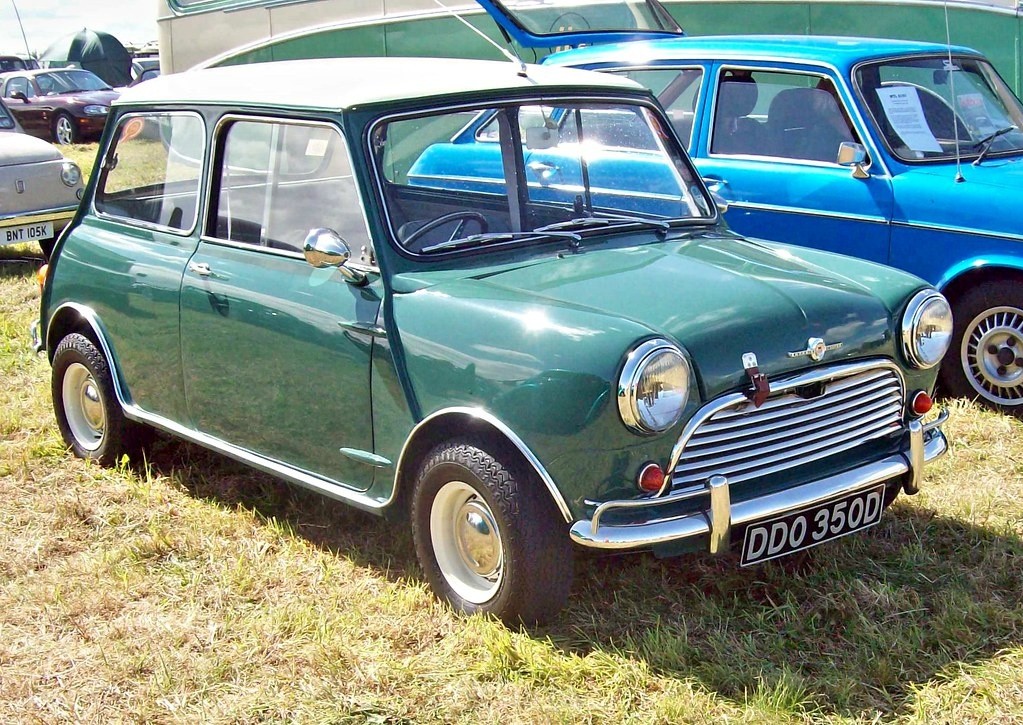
[0,68,144,145]
[31,56,954,629]
[130,57,160,82]
[407,1,1022,420]
[0,54,54,94]
[0,95,86,262]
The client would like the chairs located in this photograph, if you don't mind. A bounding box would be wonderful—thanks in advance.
[397,214,512,252]
[692,77,769,155]
[768,89,814,158]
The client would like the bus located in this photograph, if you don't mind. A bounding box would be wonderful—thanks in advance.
[155,1,1022,186]
